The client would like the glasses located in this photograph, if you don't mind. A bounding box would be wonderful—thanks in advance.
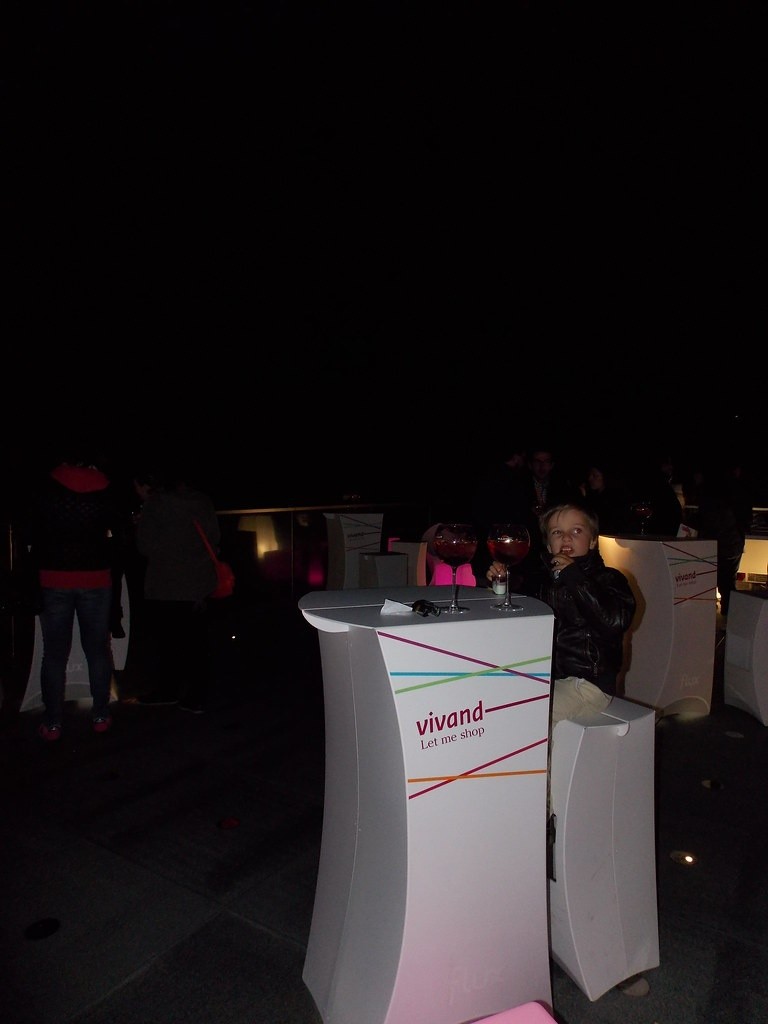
[531,457,553,464]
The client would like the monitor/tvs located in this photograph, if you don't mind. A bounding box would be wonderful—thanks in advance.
[734,535,768,585]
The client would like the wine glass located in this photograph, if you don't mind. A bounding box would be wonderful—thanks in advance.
[629,501,653,537]
[487,524,530,611]
[432,523,478,615]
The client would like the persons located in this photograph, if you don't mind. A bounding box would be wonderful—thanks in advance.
[521,449,627,595]
[669,466,743,685]
[485,501,635,701]
[30,451,221,741]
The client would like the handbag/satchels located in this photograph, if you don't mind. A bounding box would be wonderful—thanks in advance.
[208,559,235,601]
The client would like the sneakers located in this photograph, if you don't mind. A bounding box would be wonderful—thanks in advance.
[95,714,110,734]
[138,688,206,715]
[37,721,63,741]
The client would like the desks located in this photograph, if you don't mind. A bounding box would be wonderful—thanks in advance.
[298,514,768,1024]
[214,505,371,517]
